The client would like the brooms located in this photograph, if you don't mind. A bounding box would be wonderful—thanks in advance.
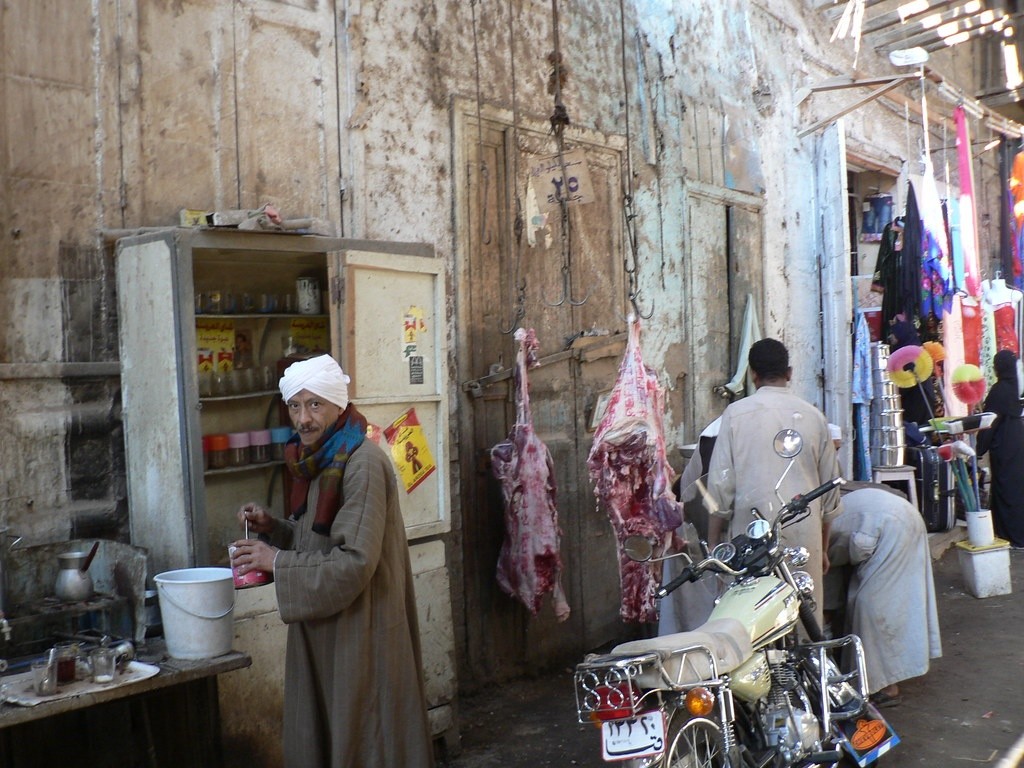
[942,411,998,511]
[928,416,975,511]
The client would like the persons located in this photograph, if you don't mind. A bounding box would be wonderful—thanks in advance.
[977,349,1023,548]
[979,277,1024,399]
[231,354,434,768]
[654,338,944,705]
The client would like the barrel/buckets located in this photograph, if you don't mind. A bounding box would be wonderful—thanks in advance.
[153,567,235,660]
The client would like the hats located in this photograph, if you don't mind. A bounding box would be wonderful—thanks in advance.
[277,354,349,410]
[829,424,842,440]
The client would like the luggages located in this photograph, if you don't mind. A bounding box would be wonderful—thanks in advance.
[908,445,957,532]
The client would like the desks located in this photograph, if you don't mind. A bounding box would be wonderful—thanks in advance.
[0,636,252,768]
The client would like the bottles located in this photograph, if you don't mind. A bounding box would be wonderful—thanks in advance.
[207,434,229,469]
[250,429,272,463]
[271,427,291,461]
[229,431,250,466]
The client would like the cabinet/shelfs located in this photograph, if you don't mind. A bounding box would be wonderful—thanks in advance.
[113,228,455,768]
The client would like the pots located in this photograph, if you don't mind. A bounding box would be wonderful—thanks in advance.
[871,340,907,468]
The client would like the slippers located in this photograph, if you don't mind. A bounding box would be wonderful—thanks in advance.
[871,693,901,709]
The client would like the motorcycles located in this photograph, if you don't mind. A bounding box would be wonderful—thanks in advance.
[574,429,901,768]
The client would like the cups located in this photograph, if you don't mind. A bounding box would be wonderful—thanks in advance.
[198,365,275,396]
[30,658,58,696]
[91,648,115,683]
[55,640,78,686]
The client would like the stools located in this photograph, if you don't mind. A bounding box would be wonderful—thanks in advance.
[873,466,919,514]
